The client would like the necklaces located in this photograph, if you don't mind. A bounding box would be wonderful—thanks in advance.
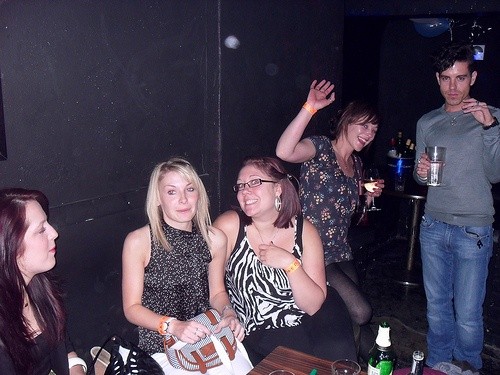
[451,117,455,126]
[254,224,279,244]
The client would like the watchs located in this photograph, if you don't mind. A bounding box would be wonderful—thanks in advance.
[164,318,176,331]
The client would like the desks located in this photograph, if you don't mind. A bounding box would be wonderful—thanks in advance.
[381,191,427,287]
[246,345,367,375]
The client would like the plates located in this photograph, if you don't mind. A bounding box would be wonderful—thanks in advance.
[393,367,445,375]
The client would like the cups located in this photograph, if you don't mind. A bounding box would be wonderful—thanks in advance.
[425,145,446,187]
[331,359,362,375]
[269,369,295,375]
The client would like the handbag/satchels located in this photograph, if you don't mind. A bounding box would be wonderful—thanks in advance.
[163,308,237,374]
[86,335,165,375]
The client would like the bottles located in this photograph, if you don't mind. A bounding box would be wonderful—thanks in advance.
[367,320,396,375]
[390,131,416,158]
[405,350,424,375]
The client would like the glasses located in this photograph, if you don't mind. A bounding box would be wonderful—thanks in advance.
[233,179,278,192]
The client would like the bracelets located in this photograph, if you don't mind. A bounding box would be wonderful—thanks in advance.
[483,117,499,130]
[159,316,169,334]
[303,102,317,116]
[284,258,301,272]
[222,306,232,316]
[69,357,87,372]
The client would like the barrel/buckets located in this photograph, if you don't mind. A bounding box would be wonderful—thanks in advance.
[388,155,415,192]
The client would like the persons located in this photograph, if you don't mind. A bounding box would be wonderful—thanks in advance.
[276,80,385,365]
[212,157,358,365]
[122,159,254,375]
[413,44,500,375]
[0,188,85,375]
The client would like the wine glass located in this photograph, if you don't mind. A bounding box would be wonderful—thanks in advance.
[362,168,381,211]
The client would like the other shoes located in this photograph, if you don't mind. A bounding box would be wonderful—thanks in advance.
[451,359,480,372]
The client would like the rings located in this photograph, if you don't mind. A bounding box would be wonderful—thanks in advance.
[195,329,198,334]
[476,101,479,105]
